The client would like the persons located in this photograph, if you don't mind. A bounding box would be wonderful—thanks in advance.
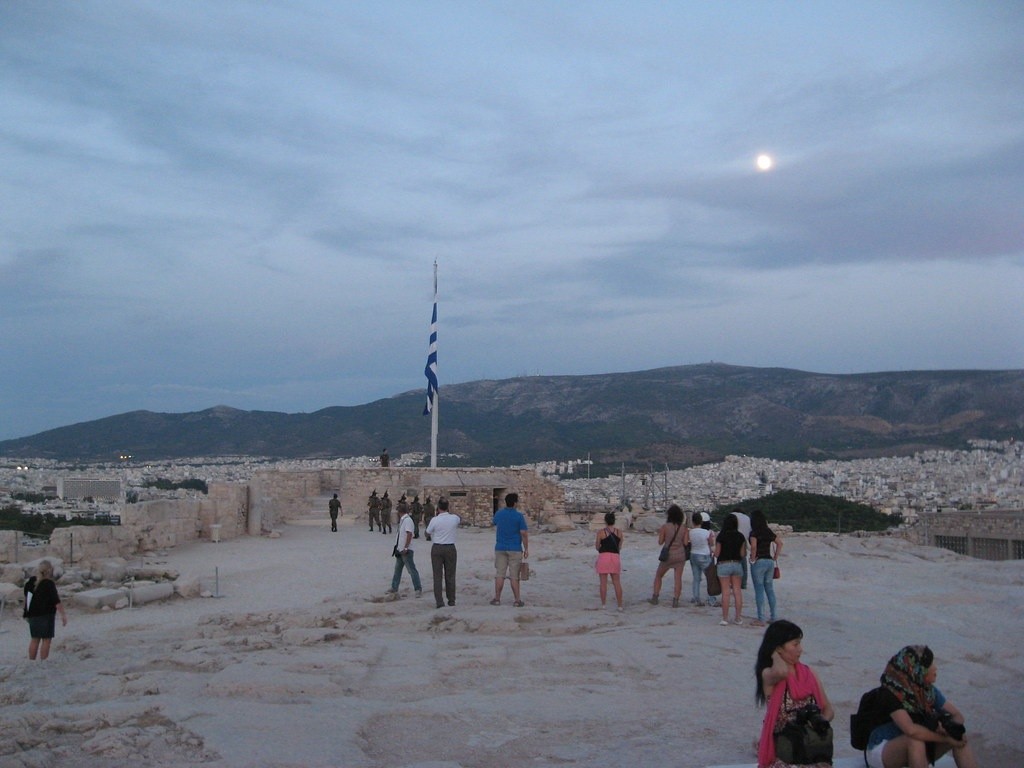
[385,505,423,598]
[755,620,835,768]
[328,494,343,531]
[731,508,752,589]
[647,504,689,607]
[867,645,977,768]
[689,513,722,607]
[425,498,460,608]
[595,513,624,609]
[381,449,390,467]
[491,493,530,607]
[367,490,393,534]
[23,560,67,660]
[396,494,449,541]
[748,511,783,626]
[713,514,746,625]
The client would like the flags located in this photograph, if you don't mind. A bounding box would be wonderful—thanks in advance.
[423,296,438,415]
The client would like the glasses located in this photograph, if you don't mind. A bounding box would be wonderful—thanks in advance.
[784,689,813,713]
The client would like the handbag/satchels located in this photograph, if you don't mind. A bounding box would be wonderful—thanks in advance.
[659,545,668,561]
[773,704,833,764]
[395,550,400,557]
[520,558,529,580]
[683,541,691,561]
[704,553,721,596]
[773,567,780,579]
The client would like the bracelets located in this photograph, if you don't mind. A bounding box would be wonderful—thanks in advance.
[750,559,756,564]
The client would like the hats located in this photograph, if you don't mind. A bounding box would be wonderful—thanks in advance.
[700,512,710,522]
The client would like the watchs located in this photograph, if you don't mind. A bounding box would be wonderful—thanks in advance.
[404,548,408,550]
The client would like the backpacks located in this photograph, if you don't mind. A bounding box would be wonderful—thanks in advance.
[851,687,880,749]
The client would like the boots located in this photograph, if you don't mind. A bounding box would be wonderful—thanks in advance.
[331,526,432,541]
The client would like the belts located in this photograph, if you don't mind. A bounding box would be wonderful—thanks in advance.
[434,543,454,545]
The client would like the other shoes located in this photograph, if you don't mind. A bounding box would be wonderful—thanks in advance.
[751,619,765,627]
[720,619,728,625]
[601,593,721,612]
[388,589,525,608]
[735,616,743,625]
[767,619,773,624]
